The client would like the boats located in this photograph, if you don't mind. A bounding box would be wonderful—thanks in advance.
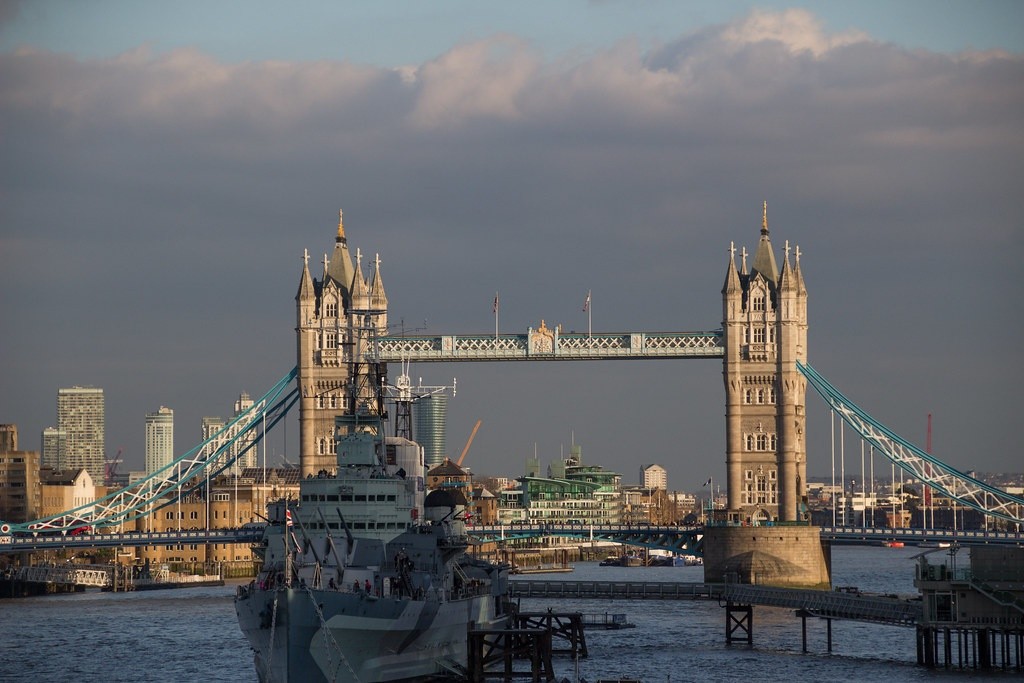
[232,308,519,683]
[563,611,636,630]
[621,555,641,567]
[133,563,225,591]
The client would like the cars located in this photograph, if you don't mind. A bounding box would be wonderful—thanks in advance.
[566,520,583,525]
[636,522,651,526]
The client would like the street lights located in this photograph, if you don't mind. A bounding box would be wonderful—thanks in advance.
[493,291,499,350]
[582,289,592,349]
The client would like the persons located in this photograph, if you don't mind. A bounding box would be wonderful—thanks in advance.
[329,577,337,590]
[365,578,372,596]
[259,579,265,590]
[249,579,256,593]
[394,552,415,572]
[471,576,476,591]
[352,579,360,593]
[416,586,423,597]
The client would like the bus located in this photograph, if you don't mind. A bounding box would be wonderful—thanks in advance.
[30,519,92,536]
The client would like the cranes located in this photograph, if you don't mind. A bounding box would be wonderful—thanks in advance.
[457,419,483,466]
[106,449,123,479]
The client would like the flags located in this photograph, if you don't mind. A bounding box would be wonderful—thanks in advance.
[286,509,301,554]
[703,478,711,486]
